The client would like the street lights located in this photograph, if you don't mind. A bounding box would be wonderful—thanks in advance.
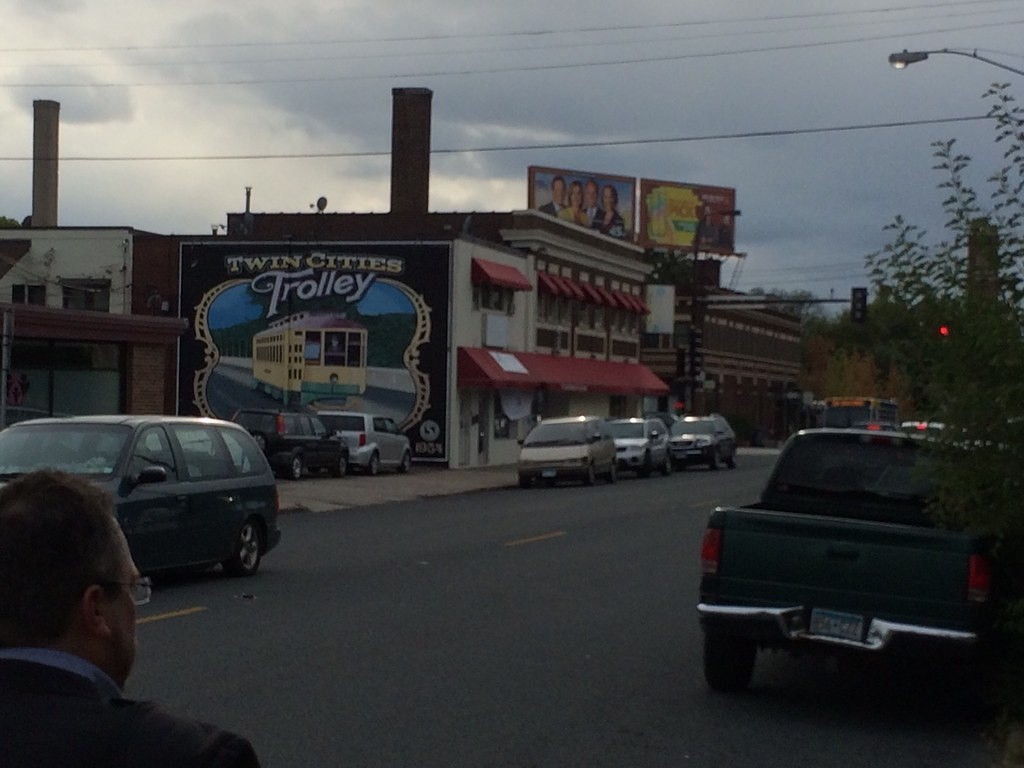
[689,211,740,413]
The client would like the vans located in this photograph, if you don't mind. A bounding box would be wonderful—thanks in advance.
[517,415,618,488]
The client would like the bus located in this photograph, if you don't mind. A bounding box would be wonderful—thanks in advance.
[822,396,898,432]
[252,311,368,411]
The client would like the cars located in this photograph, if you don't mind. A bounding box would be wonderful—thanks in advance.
[850,420,896,433]
[1,416,284,577]
[317,410,412,475]
[640,412,677,427]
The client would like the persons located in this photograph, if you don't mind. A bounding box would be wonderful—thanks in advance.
[595,185,624,239]
[540,175,567,217]
[583,181,605,228]
[699,206,732,248]
[559,180,588,225]
[0,471,260,768]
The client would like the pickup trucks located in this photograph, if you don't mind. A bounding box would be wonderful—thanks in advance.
[697,425,1002,714]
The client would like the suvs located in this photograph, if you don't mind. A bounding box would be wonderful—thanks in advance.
[604,417,670,480]
[231,409,350,481]
[669,412,738,473]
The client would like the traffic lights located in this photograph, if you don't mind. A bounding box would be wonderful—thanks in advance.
[940,323,952,337]
[851,288,868,324]
[692,327,702,376]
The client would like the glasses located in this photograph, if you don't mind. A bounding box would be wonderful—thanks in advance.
[106,577,152,608]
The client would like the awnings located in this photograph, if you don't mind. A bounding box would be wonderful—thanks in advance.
[471,258,532,291]
[541,273,649,315]
[457,347,669,395]
